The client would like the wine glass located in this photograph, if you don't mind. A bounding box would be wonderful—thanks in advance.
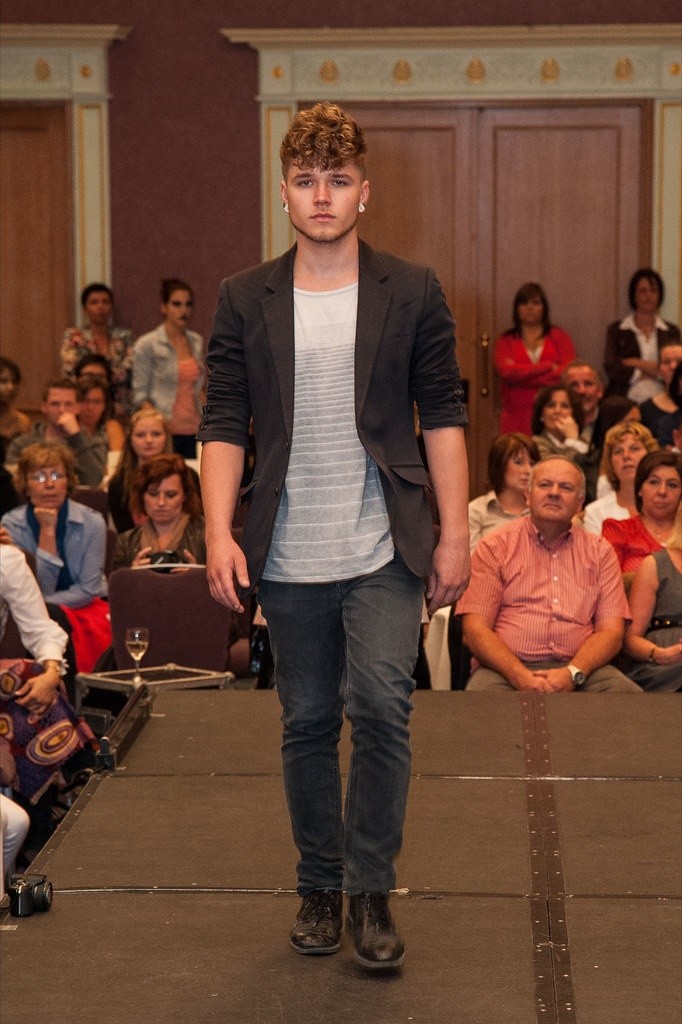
[125,626,150,683]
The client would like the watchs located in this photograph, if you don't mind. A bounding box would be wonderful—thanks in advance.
[567,664,585,686]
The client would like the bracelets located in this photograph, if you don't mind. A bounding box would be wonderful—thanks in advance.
[648,647,659,664]
[46,663,61,673]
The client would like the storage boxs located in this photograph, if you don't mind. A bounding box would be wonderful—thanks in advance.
[76,663,233,738]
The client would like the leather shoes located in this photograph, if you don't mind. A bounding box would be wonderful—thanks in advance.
[286,888,342,952]
[345,891,404,967]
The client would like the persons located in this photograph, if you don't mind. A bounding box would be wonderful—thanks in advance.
[73,353,125,483]
[603,267,682,441]
[133,277,204,459]
[108,410,201,535]
[59,284,135,418]
[1,439,112,707]
[199,103,472,969]
[0,357,33,518]
[7,379,109,485]
[0,522,74,917]
[493,282,575,436]
[82,454,240,714]
[623,500,682,694]
[248,584,431,691]
[453,455,646,694]
[468,344,682,576]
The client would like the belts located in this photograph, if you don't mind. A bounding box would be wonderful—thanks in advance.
[644,612,682,631]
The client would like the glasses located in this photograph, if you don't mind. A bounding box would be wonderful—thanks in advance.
[25,471,68,483]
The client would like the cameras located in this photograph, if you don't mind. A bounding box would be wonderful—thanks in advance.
[10,873,52,919]
[146,549,181,575]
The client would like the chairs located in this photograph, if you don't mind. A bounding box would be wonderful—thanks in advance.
[109,561,232,695]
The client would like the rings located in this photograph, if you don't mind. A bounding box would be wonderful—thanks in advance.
[464,584,468,589]
